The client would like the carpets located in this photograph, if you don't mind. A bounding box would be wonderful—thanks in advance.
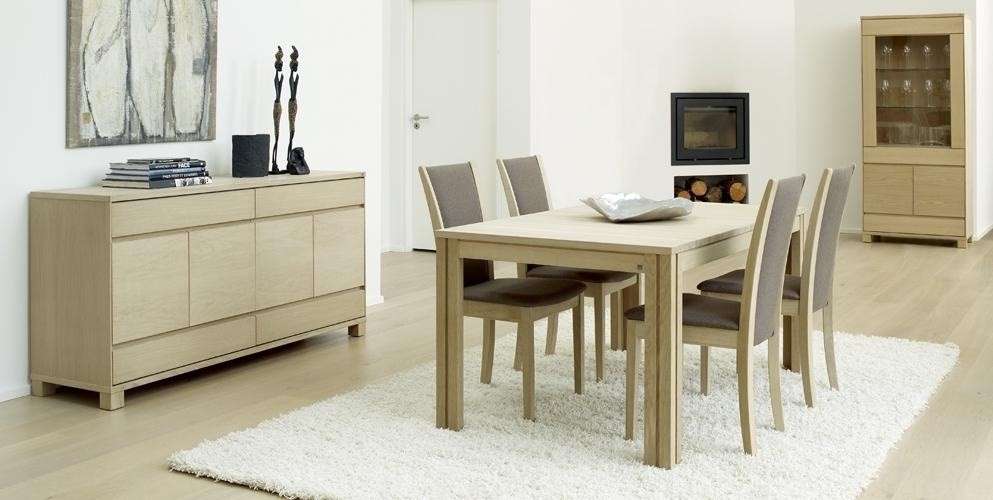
[166,305,960,499]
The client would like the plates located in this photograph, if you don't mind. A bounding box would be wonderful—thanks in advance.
[579,192,692,223]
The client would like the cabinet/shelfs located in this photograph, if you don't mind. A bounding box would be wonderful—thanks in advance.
[27,167,368,409]
[861,12,974,249]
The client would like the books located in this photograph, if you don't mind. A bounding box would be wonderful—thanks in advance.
[100,157,214,190]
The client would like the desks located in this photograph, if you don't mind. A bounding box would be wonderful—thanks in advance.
[434,200,809,470]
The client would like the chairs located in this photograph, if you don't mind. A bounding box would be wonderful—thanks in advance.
[624,173,807,455]
[498,155,638,383]
[419,160,589,421]
[696,165,852,409]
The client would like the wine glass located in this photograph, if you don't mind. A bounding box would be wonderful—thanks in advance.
[879,79,891,106]
[901,43,913,68]
[919,44,935,69]
[881,41,895,68]
[922,79,936,107]
[940,80,951,106]
[941,44,950,68]
[899,79,914,108]
